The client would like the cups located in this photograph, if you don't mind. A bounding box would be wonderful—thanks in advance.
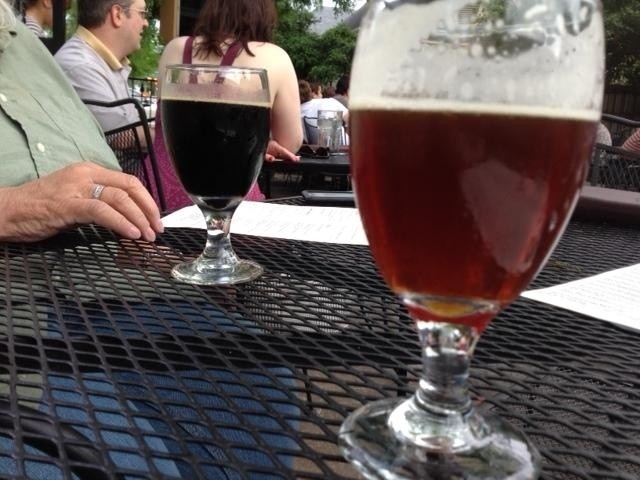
[317,109,344,154]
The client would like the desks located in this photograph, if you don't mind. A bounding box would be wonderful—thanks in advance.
[0,222,640,478]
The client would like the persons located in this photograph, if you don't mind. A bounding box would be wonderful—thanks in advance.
[299,70,351,147]
[53,0,157,167]
[151,1,304,209]
[0,0,303,480]
[17,0,71,40]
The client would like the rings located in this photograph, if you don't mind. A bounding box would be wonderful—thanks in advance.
[92,184,106,199]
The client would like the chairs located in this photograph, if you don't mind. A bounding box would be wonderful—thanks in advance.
[303,116,347,145]
[591,142,640,192]
[77,99,167,209]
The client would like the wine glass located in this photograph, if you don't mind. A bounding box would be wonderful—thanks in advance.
[160,64,273,285]
[337,1,612,479]
